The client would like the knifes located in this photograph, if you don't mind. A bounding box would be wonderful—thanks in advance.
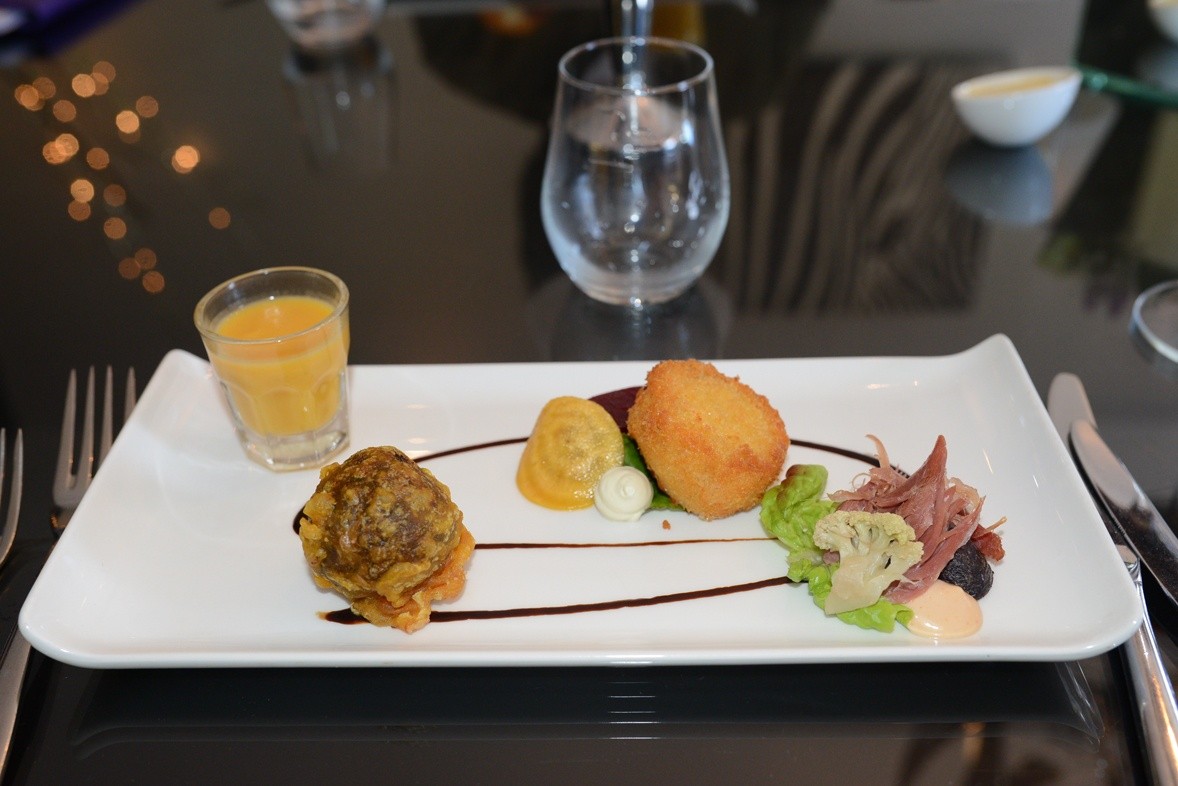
[1047,372,1178,786]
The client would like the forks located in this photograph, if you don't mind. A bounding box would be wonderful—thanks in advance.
[1,363,134,776]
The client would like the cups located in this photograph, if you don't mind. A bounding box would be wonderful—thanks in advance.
[194,264,350,472]
[540,38,730,306]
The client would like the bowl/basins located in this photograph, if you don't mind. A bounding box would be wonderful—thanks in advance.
[950,65,1083,147]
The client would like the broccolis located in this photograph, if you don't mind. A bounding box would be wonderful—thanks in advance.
[813,508,925,614]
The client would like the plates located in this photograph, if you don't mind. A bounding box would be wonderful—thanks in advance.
[18,334,1145,665]
